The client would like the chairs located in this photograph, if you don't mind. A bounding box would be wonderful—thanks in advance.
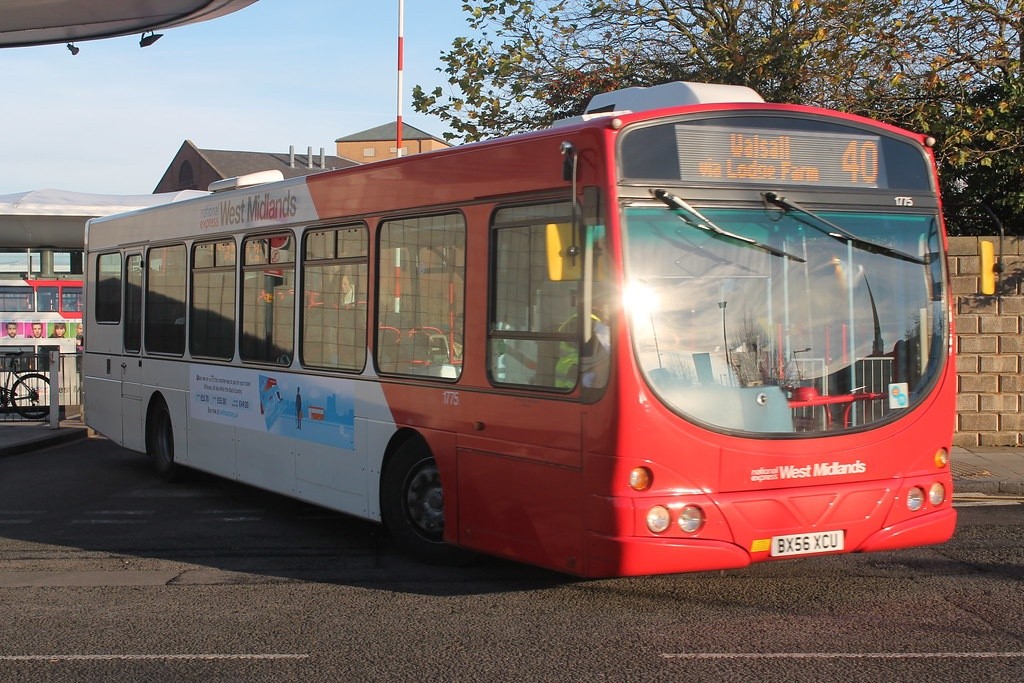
[265,285,464,372]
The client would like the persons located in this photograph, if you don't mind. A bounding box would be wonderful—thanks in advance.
[26,323,45,338]
[2,322,23,339]
[48,323,67,338]
[332,269,356,308]
[556,292,613,389]
[75,323,84,338]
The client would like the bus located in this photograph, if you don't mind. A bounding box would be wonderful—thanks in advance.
[82,80,1006,578]
[0,279,83,405]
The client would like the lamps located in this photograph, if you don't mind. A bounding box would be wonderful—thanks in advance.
[66,42,79,55]
[139,29,164,48]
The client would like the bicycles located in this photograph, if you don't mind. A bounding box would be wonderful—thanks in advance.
[0,351,50,420]
